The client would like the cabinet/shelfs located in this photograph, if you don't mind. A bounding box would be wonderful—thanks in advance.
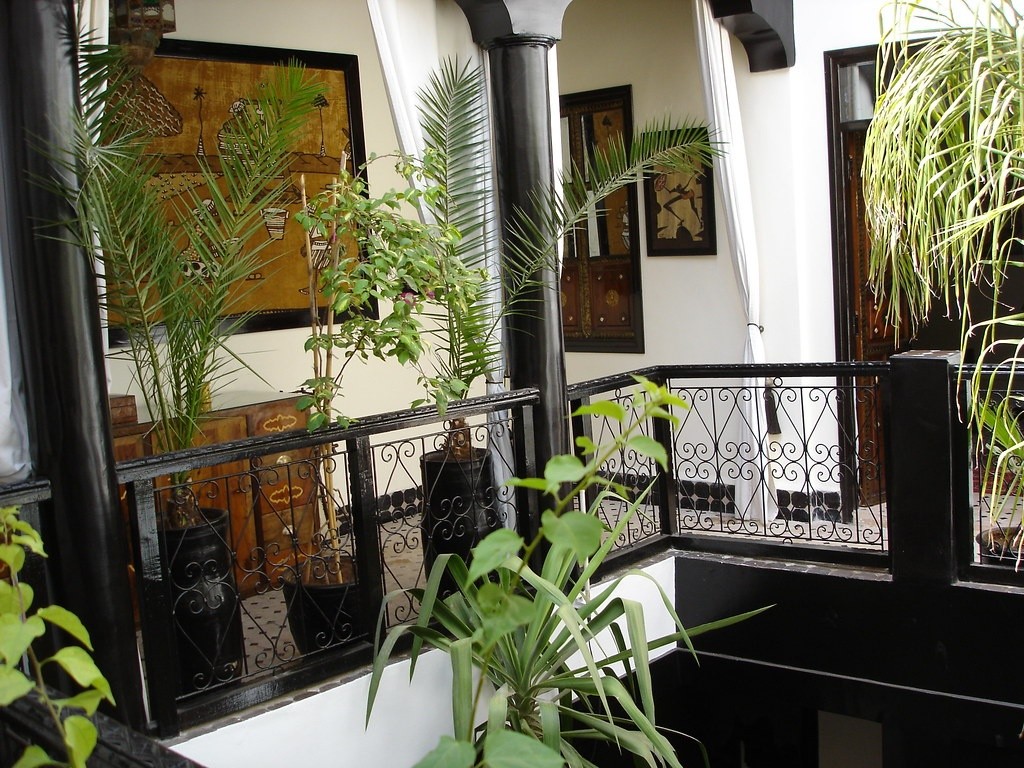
[107,387,324,632]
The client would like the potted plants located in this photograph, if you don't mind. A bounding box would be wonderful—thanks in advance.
[966,395,1024,572]
[15,1,330,699]
[417,50,733,606]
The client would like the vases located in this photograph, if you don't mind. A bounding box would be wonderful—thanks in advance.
[281,557,369,654]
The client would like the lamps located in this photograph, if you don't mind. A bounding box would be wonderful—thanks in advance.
[109,0,177,58]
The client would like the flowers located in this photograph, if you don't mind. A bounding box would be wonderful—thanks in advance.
[280,139,489,581]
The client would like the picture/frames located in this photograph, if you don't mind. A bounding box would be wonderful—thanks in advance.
[102,37,380,351]
[640,127,717,258]
